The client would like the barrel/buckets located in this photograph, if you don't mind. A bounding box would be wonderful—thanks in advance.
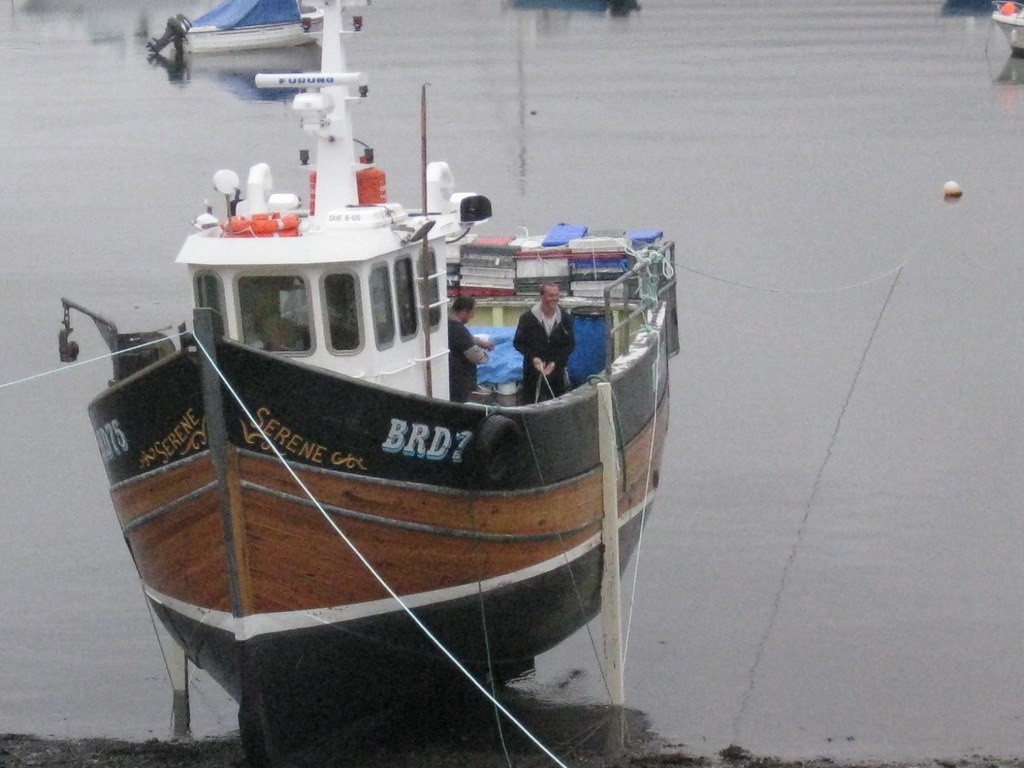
[566,306,606,387]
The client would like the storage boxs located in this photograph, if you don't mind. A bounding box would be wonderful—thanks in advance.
[447,222,665,302]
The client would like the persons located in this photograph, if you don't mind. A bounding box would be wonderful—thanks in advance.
[449,295,494,403]
[512,282,576,405]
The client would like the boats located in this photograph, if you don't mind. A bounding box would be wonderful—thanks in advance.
[988,0,1024,61]
[51,1,687,768]
[145,1,344,81]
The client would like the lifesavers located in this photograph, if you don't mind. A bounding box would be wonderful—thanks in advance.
[220,211,300,238]
